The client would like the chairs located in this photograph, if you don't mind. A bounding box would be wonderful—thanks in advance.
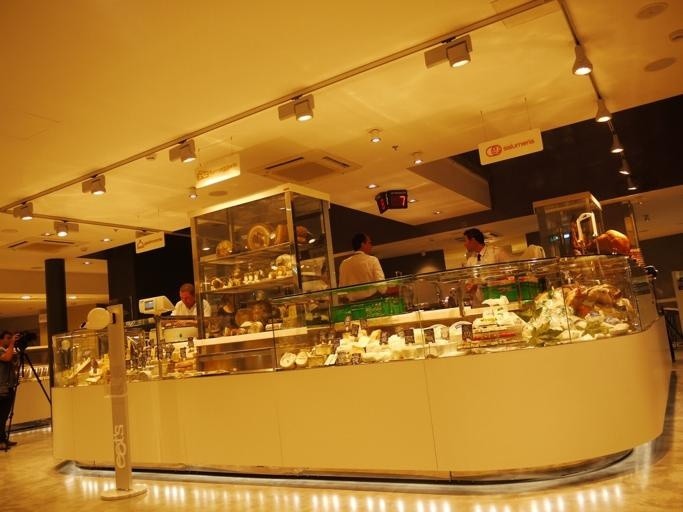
[662,268,683,363]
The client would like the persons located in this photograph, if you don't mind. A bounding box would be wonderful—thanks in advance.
[308,263,331,321]
[463,229,505,267]
[0,331,21,450]
[170,283,211,328]
[338,233,399,302]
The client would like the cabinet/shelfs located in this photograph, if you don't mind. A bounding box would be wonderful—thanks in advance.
[265,253,645,373]
[189,182,339,347]
[46,309,194,386]
[531,190,604,258]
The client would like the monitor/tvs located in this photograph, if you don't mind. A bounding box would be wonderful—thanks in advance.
[526,231,541,246]
[380,249,446,279]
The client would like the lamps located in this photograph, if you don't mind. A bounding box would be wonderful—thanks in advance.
[13,29,475,243]
[568,47,643,192]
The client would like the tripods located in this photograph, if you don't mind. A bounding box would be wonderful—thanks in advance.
[5,354,51,451]
